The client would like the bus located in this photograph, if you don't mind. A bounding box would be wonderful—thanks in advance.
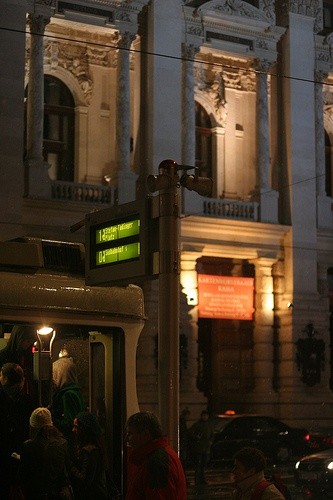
[0,232,151,500]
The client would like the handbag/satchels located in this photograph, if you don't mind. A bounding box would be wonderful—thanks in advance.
[61,484,73,499]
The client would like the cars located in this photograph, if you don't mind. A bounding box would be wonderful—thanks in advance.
[294,447,333,500]
[179,409,311,472]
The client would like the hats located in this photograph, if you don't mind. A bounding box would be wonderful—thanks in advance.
[30,407,53,428]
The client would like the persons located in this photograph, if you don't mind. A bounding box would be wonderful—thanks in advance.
[69,411,109,500]
[190,411,214,484]
[52,358,84,441]
[17,408,69,500]
[126,413,186,500]
[0,362,33,450]
[232,447,285,500]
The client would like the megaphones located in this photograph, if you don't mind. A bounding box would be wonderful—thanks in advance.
[180,173,218,199]
